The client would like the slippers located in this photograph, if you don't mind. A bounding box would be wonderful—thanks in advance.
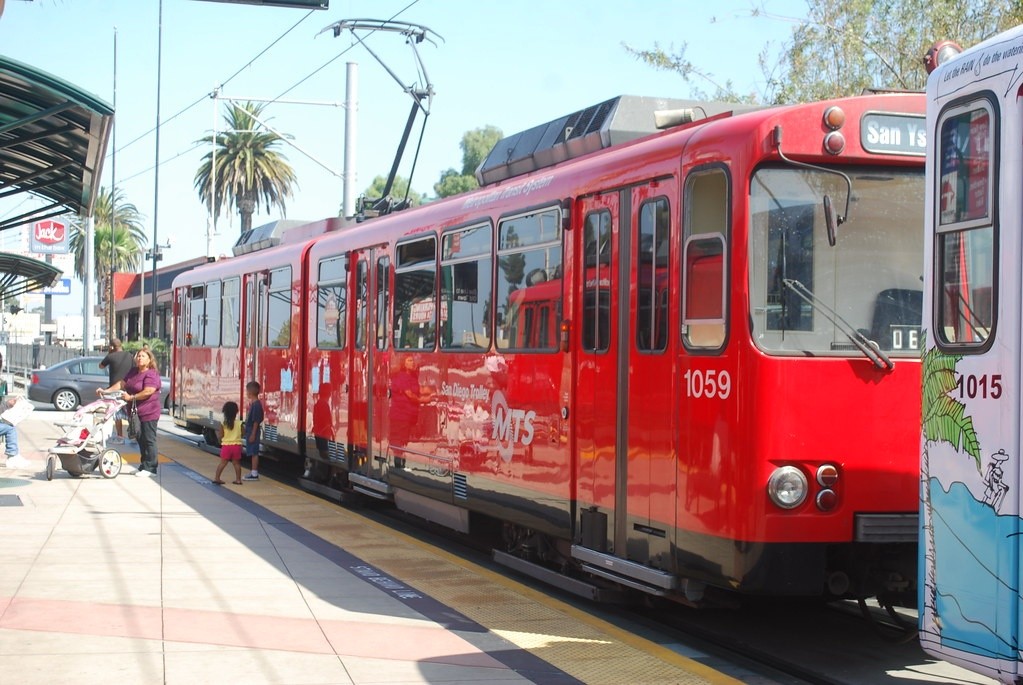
[232,481,242,484]
[212,481,224,484]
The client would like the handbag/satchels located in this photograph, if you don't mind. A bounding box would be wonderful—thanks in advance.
[127,394,141,441]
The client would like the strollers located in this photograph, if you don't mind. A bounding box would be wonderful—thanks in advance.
[46,389,129,481]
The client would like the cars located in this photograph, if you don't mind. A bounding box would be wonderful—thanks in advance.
[27,356,172,414]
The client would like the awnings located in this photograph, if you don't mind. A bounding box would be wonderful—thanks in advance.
[0,56,115,231]
[0,252,64,301]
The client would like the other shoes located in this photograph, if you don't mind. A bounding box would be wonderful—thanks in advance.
[129,438,136,443]
[107,436,125,444]
[5,458,29,469]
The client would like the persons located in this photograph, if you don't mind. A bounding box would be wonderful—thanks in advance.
[374,361,390,443]
[215,401,245,484]
[313,383,335,459]
[388,353,431,468]
[96,348,161,477]
[0,353,33,470]
[242,382,263,481]
[99,338,138,445]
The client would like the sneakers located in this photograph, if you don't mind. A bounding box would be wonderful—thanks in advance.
[135,470,157,477]
[129,469,140,474]
[242,474,258,480]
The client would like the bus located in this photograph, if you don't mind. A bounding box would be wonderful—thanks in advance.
[506,262,666,350]
[167,40,964,625]
[915,23,1023,684]
